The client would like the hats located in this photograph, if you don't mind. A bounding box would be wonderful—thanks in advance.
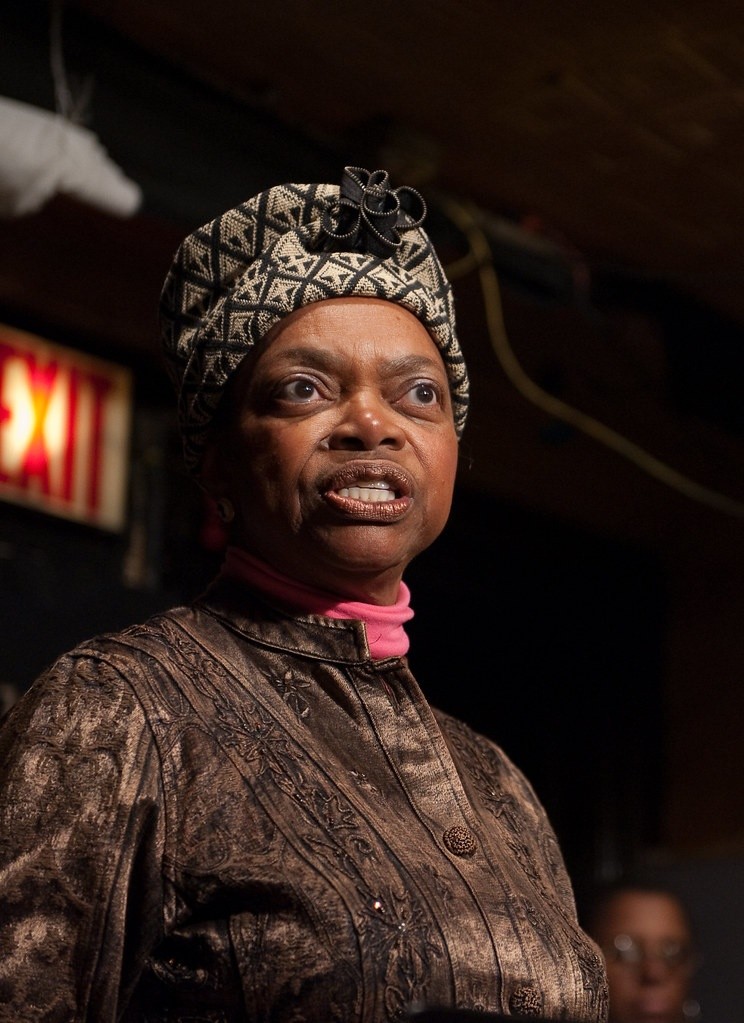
[153,167,469,442]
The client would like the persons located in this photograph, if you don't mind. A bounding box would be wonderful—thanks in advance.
[1,183,608,1022]
[597,890,695,1023]
